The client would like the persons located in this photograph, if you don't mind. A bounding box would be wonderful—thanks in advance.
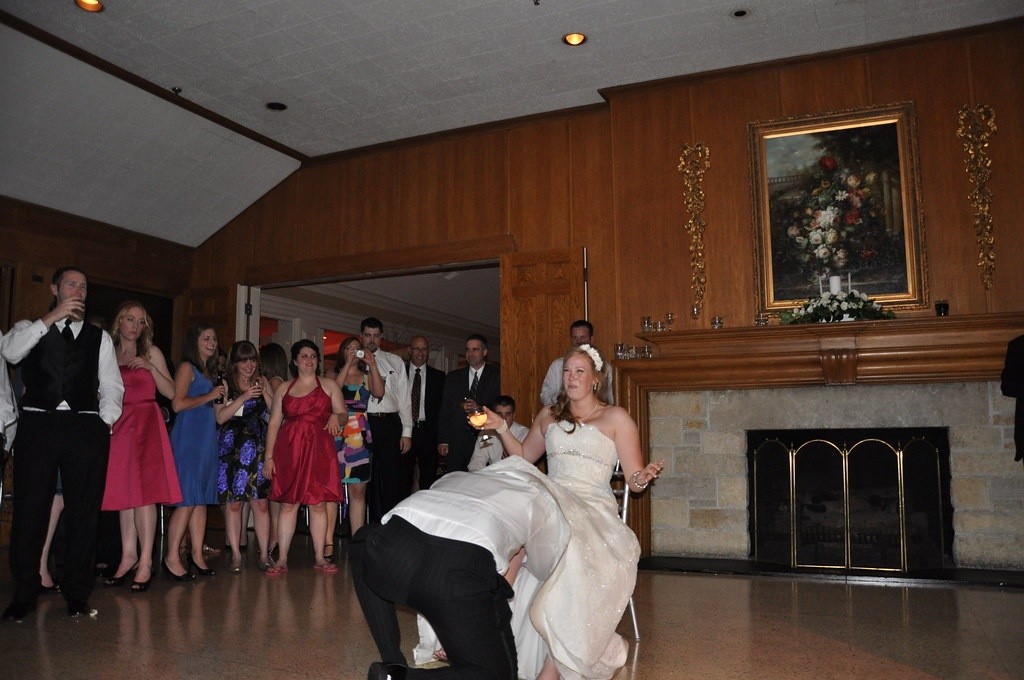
[350,467,572,680]
[0,266,126,622]
[539,320,614,407]
[467,395,530,472]
[1000,334,1024,462]
[411,344,664,680]
[102,302,500,589]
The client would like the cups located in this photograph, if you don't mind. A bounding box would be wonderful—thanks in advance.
[755,314,768,327]
[710,316,723,330]
[67,297,85,323]
[641,315,665,333]
[934,298,949,317]
[464,397,470,412]
[614,343,652,360]
[250,378,264,394]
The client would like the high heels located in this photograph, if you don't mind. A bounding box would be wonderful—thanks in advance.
[225,535,231,551]
[186,552,215,576]
[161,556,196,581]
[131,564,153,591]
[104,558,141,586]
[239,535,249,552]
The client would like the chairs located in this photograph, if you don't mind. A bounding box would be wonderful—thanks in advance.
[607,458,639,638]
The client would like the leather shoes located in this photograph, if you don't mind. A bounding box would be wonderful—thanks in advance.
[3,593,36,621]
[368,661,406,680]
[68,599,88,617]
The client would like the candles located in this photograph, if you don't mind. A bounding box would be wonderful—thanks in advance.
[829,275,841,295]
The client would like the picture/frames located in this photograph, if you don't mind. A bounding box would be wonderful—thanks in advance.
[749,101,930,317]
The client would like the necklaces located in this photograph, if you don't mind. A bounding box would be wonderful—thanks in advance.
[579,402,598,422]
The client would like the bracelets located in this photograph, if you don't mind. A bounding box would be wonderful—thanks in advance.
[496,419,508,433]
[264,457,272,460]
[633,471,649,489]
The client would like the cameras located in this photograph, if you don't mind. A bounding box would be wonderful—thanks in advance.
[353,349,365,358]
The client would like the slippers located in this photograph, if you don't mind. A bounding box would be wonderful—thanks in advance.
[314,563,338,573]
[266,566,288,576]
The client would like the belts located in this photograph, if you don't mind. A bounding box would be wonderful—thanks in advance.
[368,413,386,417]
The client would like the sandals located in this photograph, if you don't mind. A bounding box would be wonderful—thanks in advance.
[324,544,335,562]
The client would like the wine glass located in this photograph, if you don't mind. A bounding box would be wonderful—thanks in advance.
[690,303,701,330]
[467,406,493,443]
[665,312,674,331]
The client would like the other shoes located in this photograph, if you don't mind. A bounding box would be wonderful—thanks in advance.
[258,558,272,570]
[39,576,61,593]
[229,558,241,573]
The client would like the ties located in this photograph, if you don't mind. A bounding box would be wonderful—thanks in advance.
[470,372,479,401]
[61,319,74,341]
[411,368,421,421]
[502,448,509,460]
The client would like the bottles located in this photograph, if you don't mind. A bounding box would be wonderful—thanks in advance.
[214,375,224,404]
